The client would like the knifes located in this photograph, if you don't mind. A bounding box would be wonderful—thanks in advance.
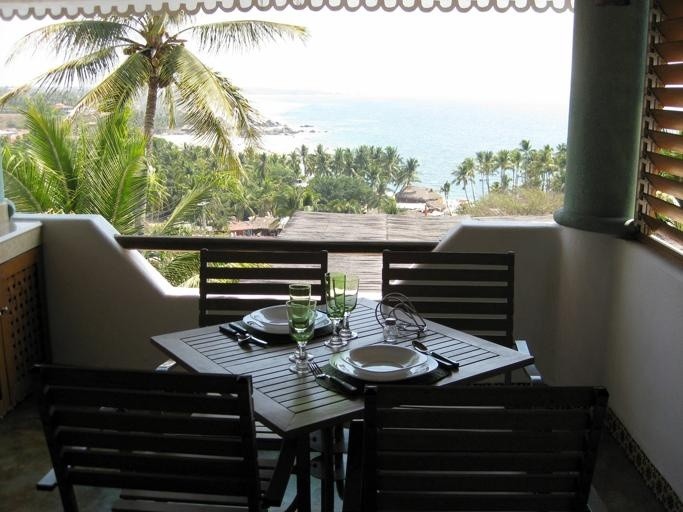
[229,322,268,346]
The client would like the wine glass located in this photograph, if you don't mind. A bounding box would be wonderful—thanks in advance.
[286,274,358,374]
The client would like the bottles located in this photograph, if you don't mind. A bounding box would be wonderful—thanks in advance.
[381,318,400,342]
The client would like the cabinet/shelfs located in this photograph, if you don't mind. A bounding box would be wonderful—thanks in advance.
[0,248,44,420]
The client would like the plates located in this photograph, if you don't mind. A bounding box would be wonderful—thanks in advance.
[242,305,332,336]
[330,346,439,382]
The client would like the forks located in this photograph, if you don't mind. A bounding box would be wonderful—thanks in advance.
[306,360,358,393]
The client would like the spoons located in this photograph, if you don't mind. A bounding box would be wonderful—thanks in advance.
[412,340,460,368]
[218,326,250,344]
